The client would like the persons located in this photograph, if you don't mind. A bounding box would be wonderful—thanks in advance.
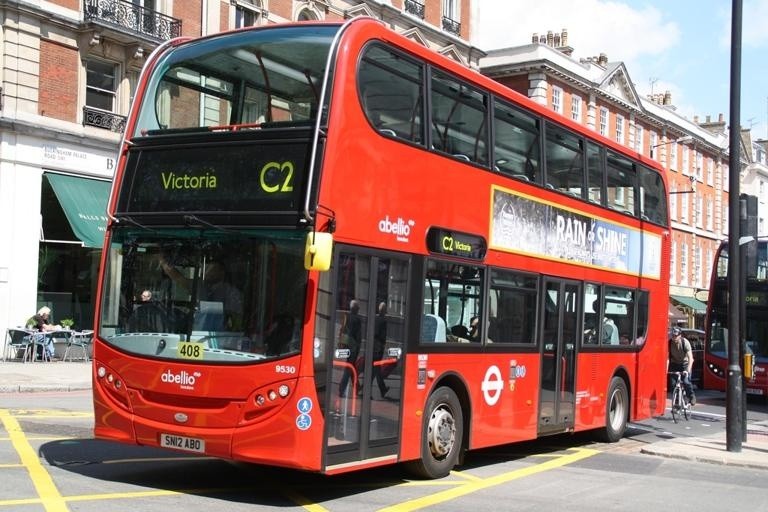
[140,289,153,302]
[336,298,362,397]
[446,315,492,344]
[666,325,698,408]
[25,306,62,360]
[584,298,644,346]
[157,252,244,332]
[369,301,390,400]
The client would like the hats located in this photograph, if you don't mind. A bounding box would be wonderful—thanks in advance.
[671,326,682,337]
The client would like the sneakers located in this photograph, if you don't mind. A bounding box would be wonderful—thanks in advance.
[690,395,696,406]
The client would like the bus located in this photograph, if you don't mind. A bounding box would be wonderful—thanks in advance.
[703,236,768,398]
[92,16,671,479]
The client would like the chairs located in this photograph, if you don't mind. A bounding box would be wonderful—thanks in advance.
[3,327,94,363]
[421,314,446,343]
[454,153,651,222]
[378,129,396,136]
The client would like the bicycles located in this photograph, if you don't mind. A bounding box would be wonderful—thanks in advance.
[667,370,694,424]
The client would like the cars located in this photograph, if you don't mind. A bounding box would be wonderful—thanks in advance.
[668,328,704,386]
